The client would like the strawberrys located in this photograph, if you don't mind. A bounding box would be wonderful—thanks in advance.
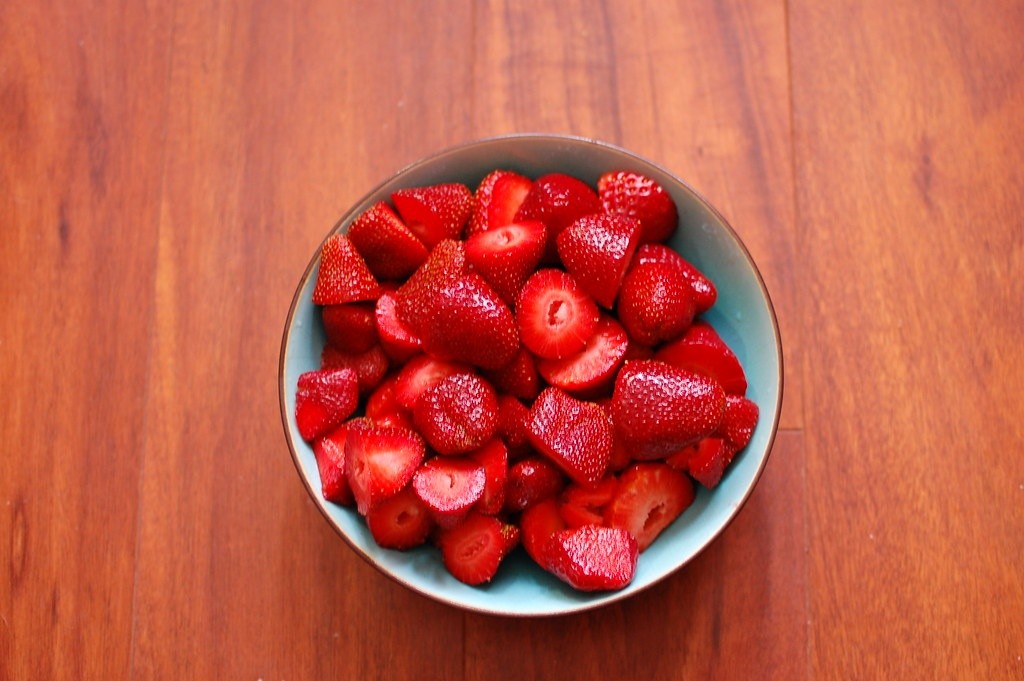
[294,168,759,591]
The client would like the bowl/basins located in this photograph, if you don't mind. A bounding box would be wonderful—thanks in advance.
[277,135,785,620]
